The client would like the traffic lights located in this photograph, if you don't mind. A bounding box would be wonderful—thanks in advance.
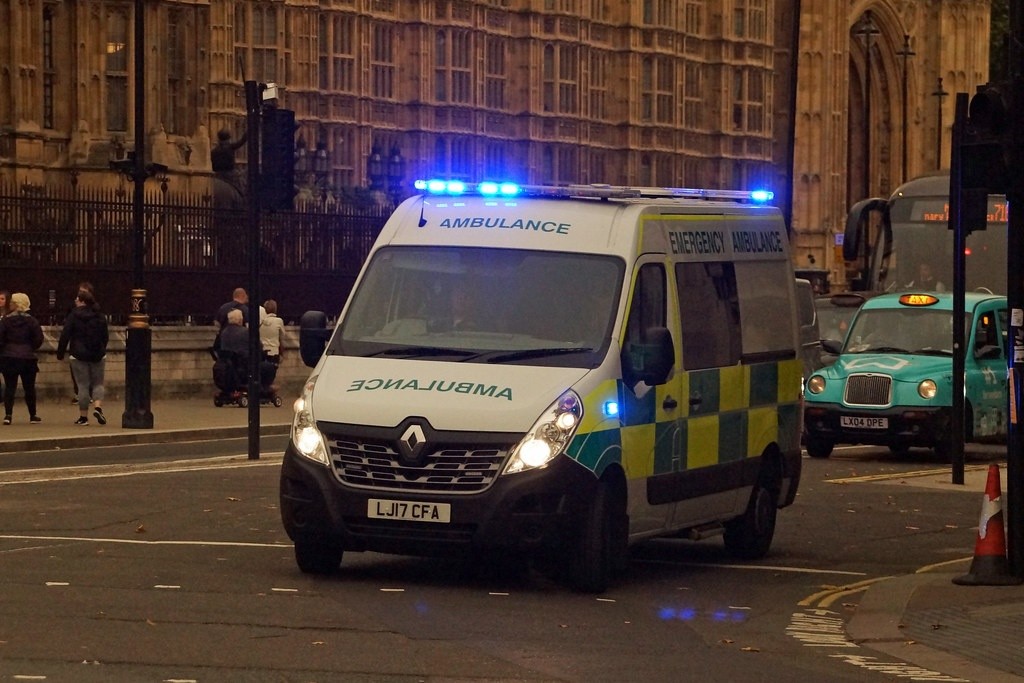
[257,109,299,209]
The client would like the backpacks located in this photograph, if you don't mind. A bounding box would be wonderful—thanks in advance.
[209,347,241,390]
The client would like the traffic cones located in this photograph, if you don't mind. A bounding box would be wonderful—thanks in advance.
[952,462,1023,586]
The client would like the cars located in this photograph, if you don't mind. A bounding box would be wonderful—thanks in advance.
[795,275,821,373]
[814,292,874,364]
[806,287,1007,462]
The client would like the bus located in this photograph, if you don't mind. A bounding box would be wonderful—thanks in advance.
[840,174,1008,295]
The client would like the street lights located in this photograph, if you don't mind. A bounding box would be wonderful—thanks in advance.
[854,8,880,194]
[931,74,949,170]
[894,34,918,184]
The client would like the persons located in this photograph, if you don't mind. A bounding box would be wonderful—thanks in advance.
[0,291,43,423]
[214,288,285,399]
[57,283,108,425]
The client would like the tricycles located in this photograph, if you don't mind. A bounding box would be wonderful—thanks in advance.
[205,346,282,408]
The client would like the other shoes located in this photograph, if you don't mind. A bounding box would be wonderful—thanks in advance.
[3,415,12,424]
[30,416,41,421]
[90,399,94,403]
[71,395,80,405]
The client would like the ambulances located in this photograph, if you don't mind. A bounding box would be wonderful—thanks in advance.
[277,178,807,594]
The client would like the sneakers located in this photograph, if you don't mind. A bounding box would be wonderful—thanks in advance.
[93,407,107,424]
[74,416,89,426]
